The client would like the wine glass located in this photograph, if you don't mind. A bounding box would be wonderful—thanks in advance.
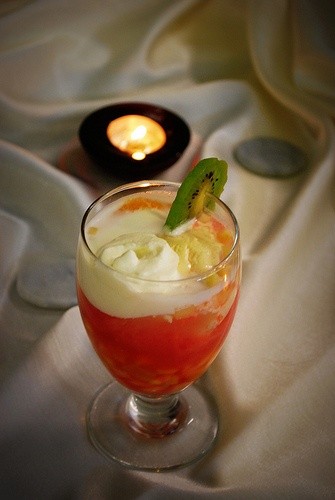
[76,180,243,474]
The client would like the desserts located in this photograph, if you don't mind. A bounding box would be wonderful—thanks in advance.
[76,195,239,400]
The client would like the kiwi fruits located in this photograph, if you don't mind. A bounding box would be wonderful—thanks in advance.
[165,157,228,230]
